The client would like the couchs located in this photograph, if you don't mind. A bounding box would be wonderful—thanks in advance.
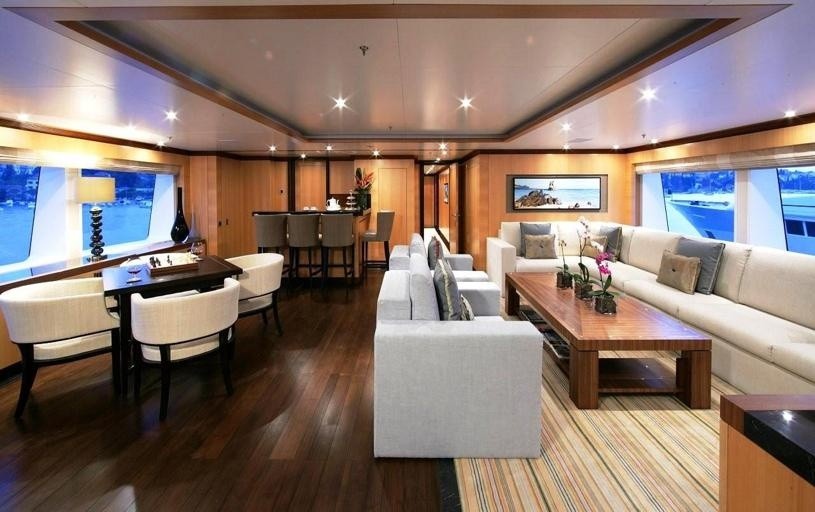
[483,218,815,397]
[387,243,489,282]
[372,267,545,461]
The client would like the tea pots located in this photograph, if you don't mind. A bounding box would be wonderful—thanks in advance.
[326,197,339,209]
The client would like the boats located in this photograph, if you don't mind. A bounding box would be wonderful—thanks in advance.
[668,190,815,256]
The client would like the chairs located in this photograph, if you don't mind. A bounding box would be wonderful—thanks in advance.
[224,251,286,334]
[252,211,292,273]
[130,276,242,423]
[0,274,123,420]
[286,212,322,299]
[320,212,357,299]
[428,233,443,269]
[361,210,397,279]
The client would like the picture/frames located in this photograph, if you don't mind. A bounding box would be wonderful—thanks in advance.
[511,176,602,211]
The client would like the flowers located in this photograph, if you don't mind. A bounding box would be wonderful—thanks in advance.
[352,168,374,190]
[596,251,619,296]
[574,215,597,278]
[556,235,571,271]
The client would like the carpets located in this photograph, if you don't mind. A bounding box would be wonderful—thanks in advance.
[435,291,743,512]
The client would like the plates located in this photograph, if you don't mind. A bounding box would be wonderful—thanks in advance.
[326,208,342,211]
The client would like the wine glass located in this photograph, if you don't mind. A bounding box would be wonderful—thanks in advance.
[126,256,144,283]
[191,242,208,261]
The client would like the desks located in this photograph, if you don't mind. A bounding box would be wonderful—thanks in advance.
[100,253,245,396]
[717,392,815,512]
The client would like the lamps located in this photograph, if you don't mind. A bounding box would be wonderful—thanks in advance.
[73,175,119,258]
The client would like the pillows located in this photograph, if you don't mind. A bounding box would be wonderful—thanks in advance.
[459,293,476,323]
[582,235,608,258]
[672,235,727,297]
[655,248,704,296]
[432,258,463,322]
[519,221,553,255]
[523,232,558,260]
[597,225,623,264]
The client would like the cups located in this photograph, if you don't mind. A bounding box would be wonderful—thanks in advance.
[303,207,316,211]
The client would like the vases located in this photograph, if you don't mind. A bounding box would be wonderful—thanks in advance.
[356,190,369,211]
[169,185,191,244]
[595,292,620,315]
[556,270,573,291]
[574,282,593,300]
